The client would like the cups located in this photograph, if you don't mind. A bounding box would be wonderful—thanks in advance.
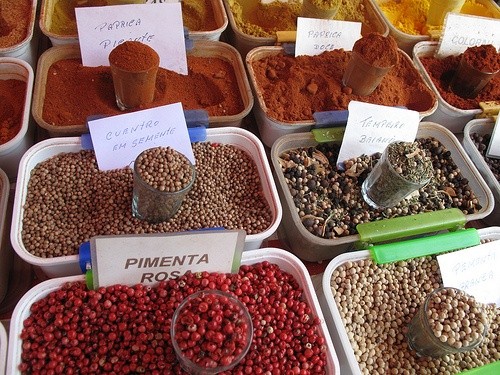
[170,290,253,375]
[429,0,465,26]
[361,140,432,209]
[408,287,486,357]
[342,37,399,95]
[451,51,500,100]
[132,146,196,219]
[109,49,160,111]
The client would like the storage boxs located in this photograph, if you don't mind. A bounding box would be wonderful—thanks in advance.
[0,0,500,375]
